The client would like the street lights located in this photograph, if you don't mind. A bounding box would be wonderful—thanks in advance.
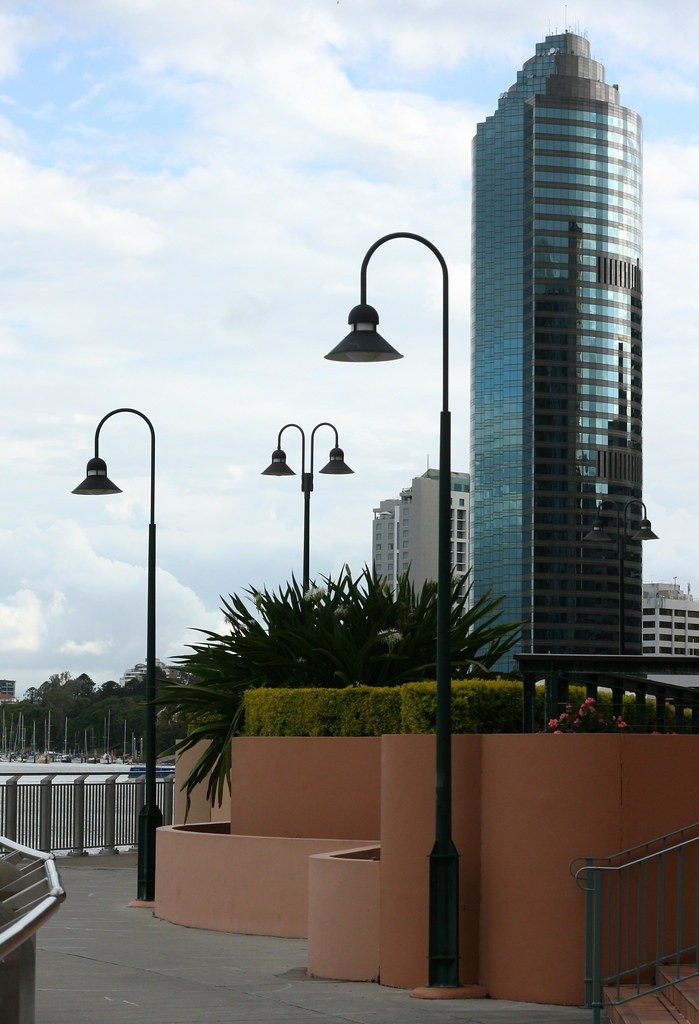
[323,233,486,998]
[581,499,660,655]
[259,424,356,604]
[69,408,165,908]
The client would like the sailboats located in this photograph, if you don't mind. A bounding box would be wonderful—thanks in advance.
[0,709,143,764]
